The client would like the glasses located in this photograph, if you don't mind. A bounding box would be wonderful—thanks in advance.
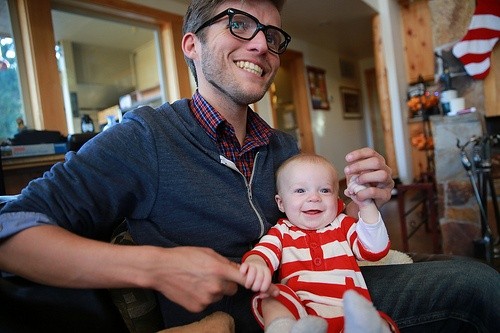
[194,7,291,55]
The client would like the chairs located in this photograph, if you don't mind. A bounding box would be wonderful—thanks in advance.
[68,132,166,333]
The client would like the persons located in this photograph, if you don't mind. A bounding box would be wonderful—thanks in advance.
[239,153,400,333]
[1,1,500,333]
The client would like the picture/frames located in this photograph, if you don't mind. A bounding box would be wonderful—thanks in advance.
[340,86,364,120]
